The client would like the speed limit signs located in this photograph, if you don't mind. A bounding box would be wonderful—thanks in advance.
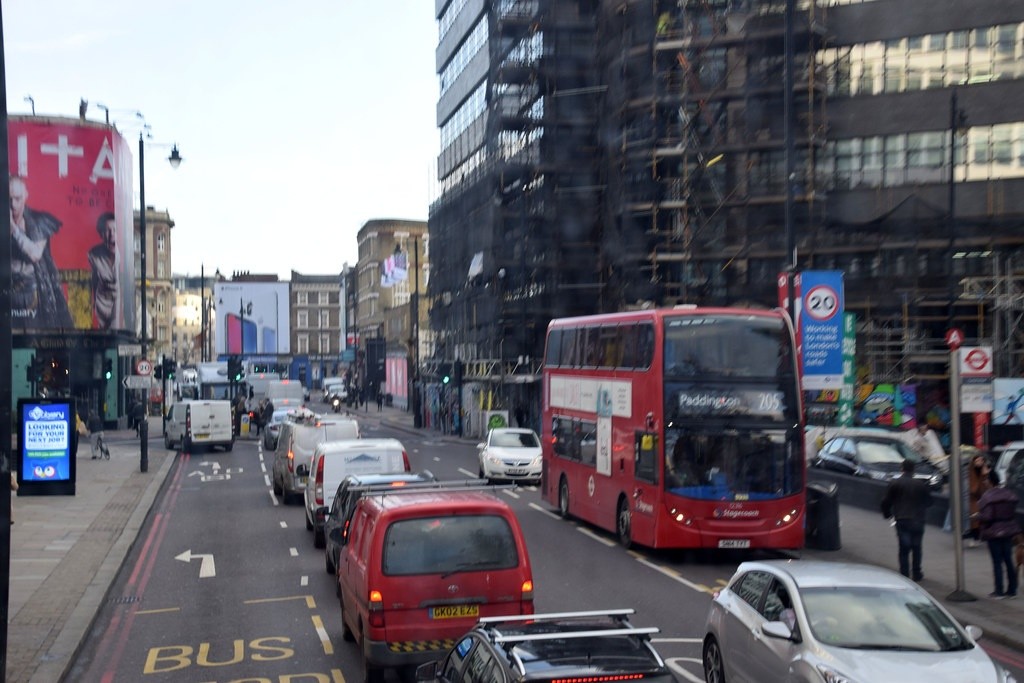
[804,285,839,318]
[136,361,152,375]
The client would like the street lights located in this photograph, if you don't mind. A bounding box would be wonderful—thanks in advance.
[393,234,422,429]
[138,131,184,473]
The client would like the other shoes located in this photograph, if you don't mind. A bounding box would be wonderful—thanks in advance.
[1005,592,1019,598]
[989,592,1005,599]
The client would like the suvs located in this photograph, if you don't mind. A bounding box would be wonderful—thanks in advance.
[414,607,680,683]
[317,468,443,573]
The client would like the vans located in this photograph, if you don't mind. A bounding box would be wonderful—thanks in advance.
[296,436,413,549]
[272,412,361,505]
[325,489,536,683]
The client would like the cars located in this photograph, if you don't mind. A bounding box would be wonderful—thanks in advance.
[249,372,351,450]
[702,559,1021,683]
[476,427,543,485]
[807,433,948,517]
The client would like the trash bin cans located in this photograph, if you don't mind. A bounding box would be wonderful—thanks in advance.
[385,393,394,407]
[805,480,844,551]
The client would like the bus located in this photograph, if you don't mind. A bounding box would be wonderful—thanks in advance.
[541,306,809,562]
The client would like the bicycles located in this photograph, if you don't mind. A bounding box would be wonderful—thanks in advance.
[89,431,111,461]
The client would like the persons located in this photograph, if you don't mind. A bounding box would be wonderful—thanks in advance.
[881,458,934,582]
[377,390,385,412]
[129,398,147,438]
[9,176,75,328]
[10,474,19,525]
[254,398,274,439]
[75,409,81,453]
[907,420,951,475]
[331,394,341,410]
[959,454,1024,600]
[87,213,116,329]
[513,402,525,428]
[86,409,109,459]
[352,386,365,407]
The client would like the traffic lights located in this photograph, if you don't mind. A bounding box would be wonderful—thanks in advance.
[163,355,174,380]
[253,364,267,373]
[440,364,450,384]
[229,358,242,381]
[103,358,112,381]
[154,364,161,380]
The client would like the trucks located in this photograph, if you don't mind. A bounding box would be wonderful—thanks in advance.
[163,399,237,454]
